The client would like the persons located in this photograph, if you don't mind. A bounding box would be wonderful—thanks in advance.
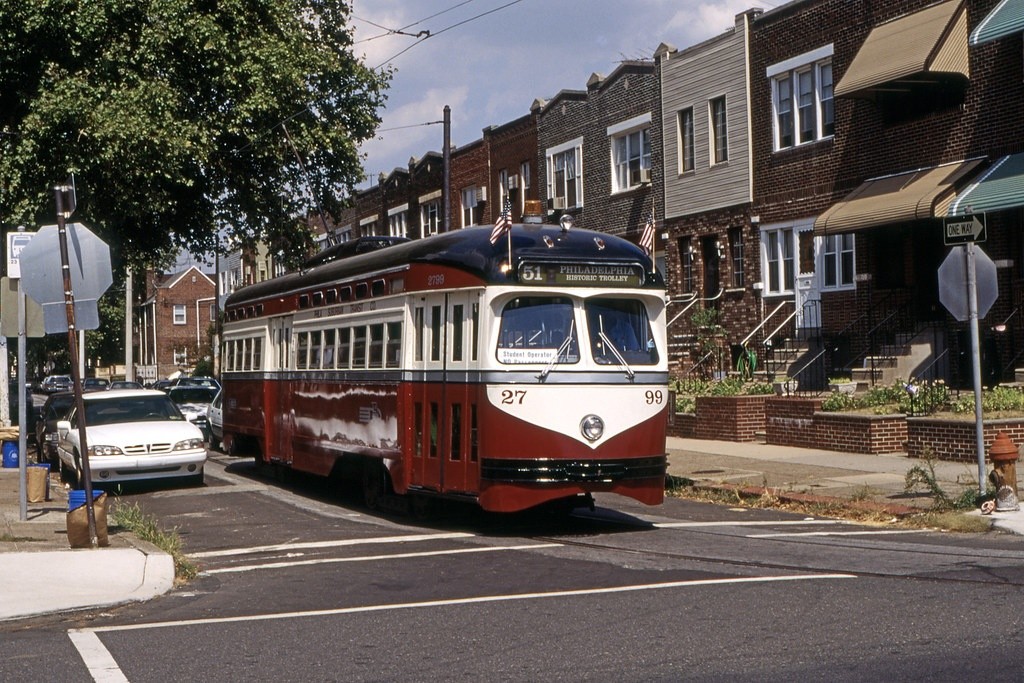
[600,306,639,351]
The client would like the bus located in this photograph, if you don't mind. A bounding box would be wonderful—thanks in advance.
[219,199,672,517]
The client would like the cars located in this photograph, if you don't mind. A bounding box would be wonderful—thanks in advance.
[36,391,98,468]
[207,387,236,453]
[26,375,222,394]
[54,388,209,492]
[163,386,218,432]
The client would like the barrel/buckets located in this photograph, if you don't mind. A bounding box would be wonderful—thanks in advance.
[68,490,104,512]
[27,463,51,500]
[2,441,19,468]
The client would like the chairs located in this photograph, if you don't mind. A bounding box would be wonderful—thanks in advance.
[143,400,168,420]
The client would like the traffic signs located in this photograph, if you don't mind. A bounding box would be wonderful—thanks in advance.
[943,211,987,246]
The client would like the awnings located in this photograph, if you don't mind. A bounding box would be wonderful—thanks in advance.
[946,154,1024,218]
[834,0,967,99]
[968,0,1024,48]
[813,156,986,236]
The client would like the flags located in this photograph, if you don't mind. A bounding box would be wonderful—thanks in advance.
[639,214,654,249]
[490,198,511,243]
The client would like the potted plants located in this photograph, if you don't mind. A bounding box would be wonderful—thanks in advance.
[772,377,799,396]
[828,377,857,397]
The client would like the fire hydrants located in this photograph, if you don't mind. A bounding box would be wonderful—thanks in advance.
[988,434,1019,512]
